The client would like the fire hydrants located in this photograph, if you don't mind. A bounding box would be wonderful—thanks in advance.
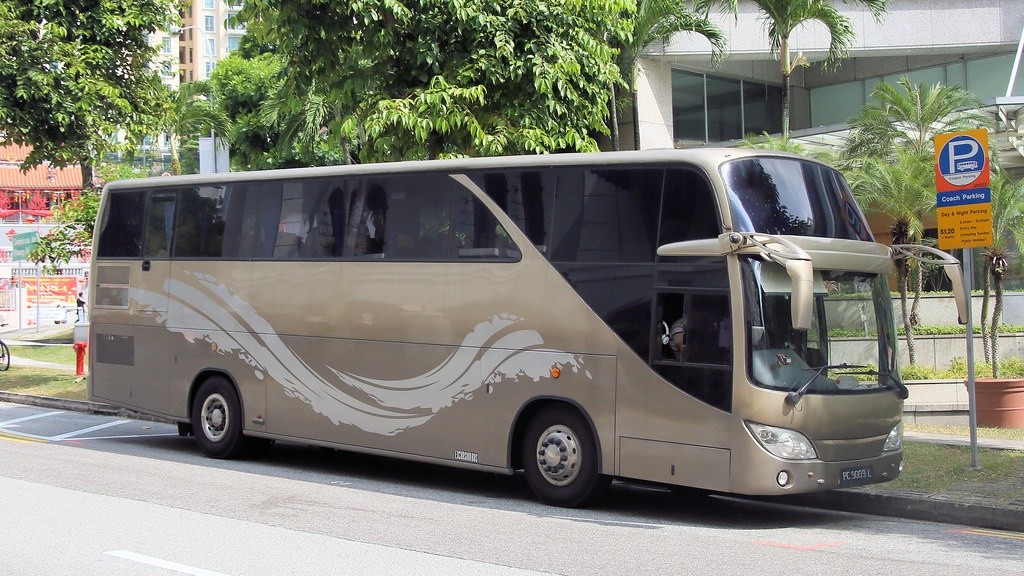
[72,339,88,376]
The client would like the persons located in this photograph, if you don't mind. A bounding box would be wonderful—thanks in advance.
[54,305,69,324]
[74,293,88,323]
[670,314,687,358]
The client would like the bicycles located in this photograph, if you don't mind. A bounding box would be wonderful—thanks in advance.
[0,323,12,371]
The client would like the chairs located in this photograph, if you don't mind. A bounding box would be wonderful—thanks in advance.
[264,226,336,258]
[382,232,463,260]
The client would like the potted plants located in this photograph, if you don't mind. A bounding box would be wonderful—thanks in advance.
[925,166,1024,430]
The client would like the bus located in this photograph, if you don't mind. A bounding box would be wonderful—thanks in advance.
[84,145,972,509]
